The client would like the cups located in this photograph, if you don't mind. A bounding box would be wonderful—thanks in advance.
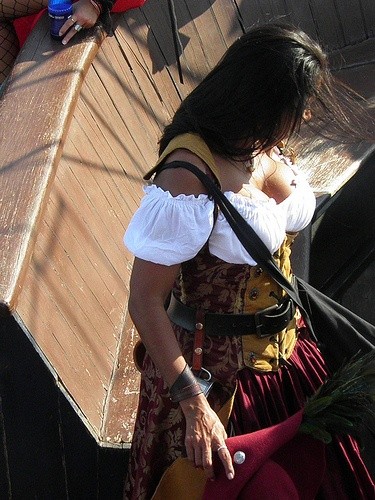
[47,0,74,42]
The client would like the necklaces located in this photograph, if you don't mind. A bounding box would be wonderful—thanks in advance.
[231,149,255,172]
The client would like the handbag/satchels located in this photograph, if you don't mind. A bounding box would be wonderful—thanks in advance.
[295,274,375,370]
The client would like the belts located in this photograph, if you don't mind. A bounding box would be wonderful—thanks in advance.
[166,291,297,336]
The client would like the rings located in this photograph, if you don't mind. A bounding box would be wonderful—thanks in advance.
[67,15,75,24]
[73,24,82,32]
[217,446,226,451]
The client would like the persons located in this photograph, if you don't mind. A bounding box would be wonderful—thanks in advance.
[126,24,327,500]
[148,340,375,500]
[0,0,145,46]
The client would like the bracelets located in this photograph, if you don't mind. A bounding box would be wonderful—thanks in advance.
[169,362,203,403]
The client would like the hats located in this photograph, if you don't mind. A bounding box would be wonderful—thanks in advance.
[151,350,375,500]
